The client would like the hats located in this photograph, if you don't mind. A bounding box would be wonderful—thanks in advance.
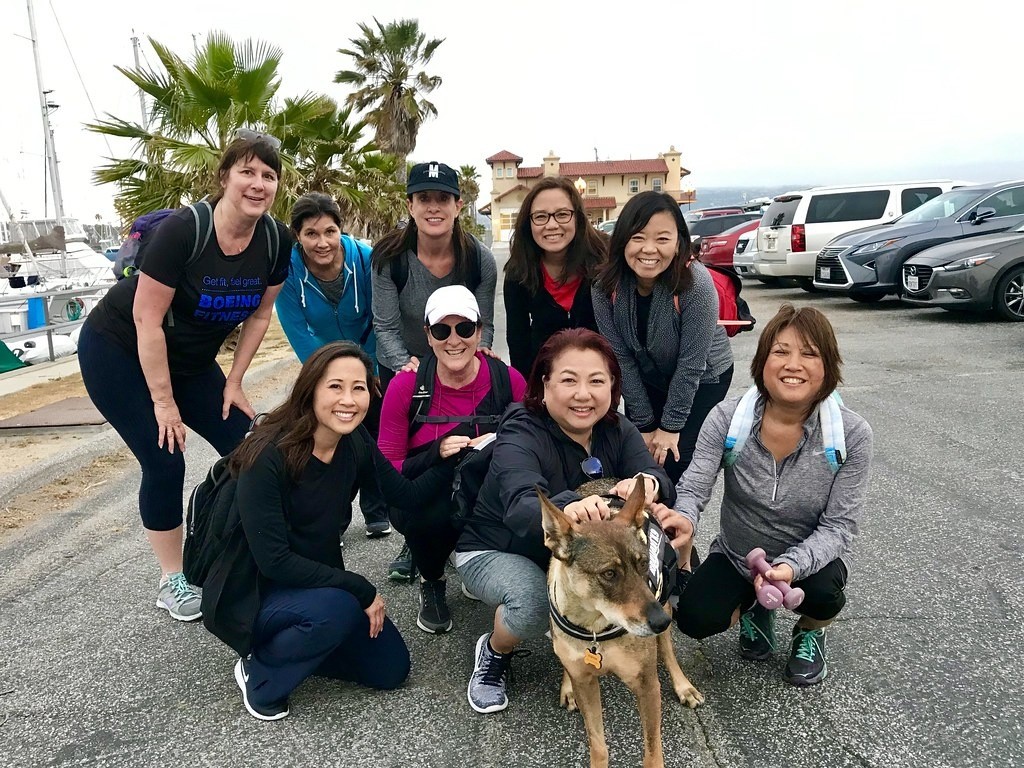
[423,284,481,326]
[407,161,461,202]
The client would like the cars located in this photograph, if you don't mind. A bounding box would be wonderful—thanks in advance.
[592,181,975,295]
[898,220,1023,320]
[812,180,1024,302]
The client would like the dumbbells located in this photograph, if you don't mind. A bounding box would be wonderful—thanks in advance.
[744,546,806,611]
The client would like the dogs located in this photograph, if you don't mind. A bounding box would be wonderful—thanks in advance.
[535,471,706,768]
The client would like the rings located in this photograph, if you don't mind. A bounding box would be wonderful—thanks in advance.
[250,403,252,407]
[662,448,667,451]
[166,429,174,432]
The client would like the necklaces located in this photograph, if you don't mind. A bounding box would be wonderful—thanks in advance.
[220,198,255,252]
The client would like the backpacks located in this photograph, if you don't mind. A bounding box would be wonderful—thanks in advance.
[182,412,271,589]
[449,434,509,545]
[113,193,279,285]
[611,253,756,338]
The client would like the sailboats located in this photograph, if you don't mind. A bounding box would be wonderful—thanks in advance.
[0,0,201,374]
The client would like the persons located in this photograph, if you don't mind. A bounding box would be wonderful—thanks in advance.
[274,191,392,539]
[460,329,677,714]
[503,177,612,383]
[378,285,528,634]
[369,162,498,579]
[79,129,293,621]
[645,304,872,687]
[200,341,493,722]
[592,190,734,572]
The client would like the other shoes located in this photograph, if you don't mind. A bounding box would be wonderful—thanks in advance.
[461,581,482,601]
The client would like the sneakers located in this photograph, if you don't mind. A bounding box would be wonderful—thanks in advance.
[671,566,695,621]
[416,573,452,634]
[467,632,508,713]
[234,653,290,721]
[155,572,203,622]
[737,601,777,660]
[387,541,419,579]
[782,622,828,685]
[365,521,392,538]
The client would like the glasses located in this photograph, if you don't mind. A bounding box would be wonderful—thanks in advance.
[234,127,282,149]
[428,320,477,341]
[580,454,603,481]
[529,208,577,226]
